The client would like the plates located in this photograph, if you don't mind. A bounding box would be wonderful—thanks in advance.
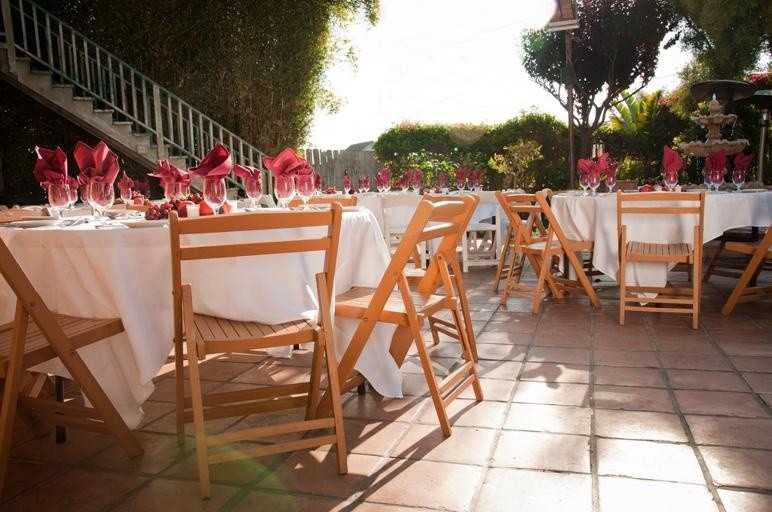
[9,216,60,228]
[741,189,768,193]
[121,220,168,228]
[343,206,364,212]
[558,192,581,196]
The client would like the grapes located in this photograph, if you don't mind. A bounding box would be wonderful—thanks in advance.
[146,193,201,220]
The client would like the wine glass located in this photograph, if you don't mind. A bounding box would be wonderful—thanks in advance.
[576,169,746,196]
[314,177,482,195]
[45,176,314,218]
